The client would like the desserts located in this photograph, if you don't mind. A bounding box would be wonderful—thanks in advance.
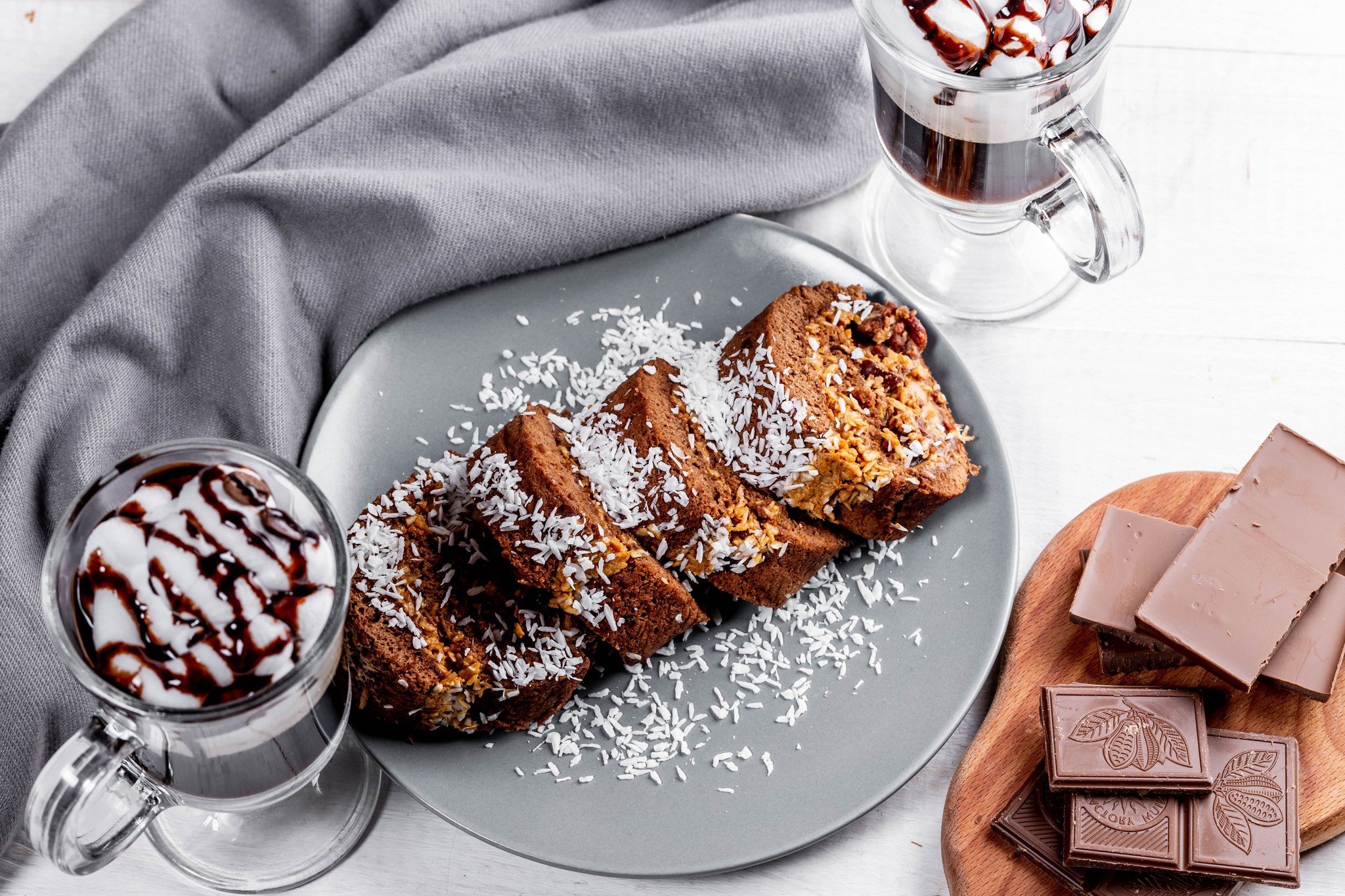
[989,422,1344,896]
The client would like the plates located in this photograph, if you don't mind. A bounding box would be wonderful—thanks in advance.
[298,210,1020,880]
[941,470,1345,896]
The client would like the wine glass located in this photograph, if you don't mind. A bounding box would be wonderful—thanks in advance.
[846,0,1146,327]
[24,436,387,893]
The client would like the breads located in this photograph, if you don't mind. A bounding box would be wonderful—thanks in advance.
[342,278,978,740]
